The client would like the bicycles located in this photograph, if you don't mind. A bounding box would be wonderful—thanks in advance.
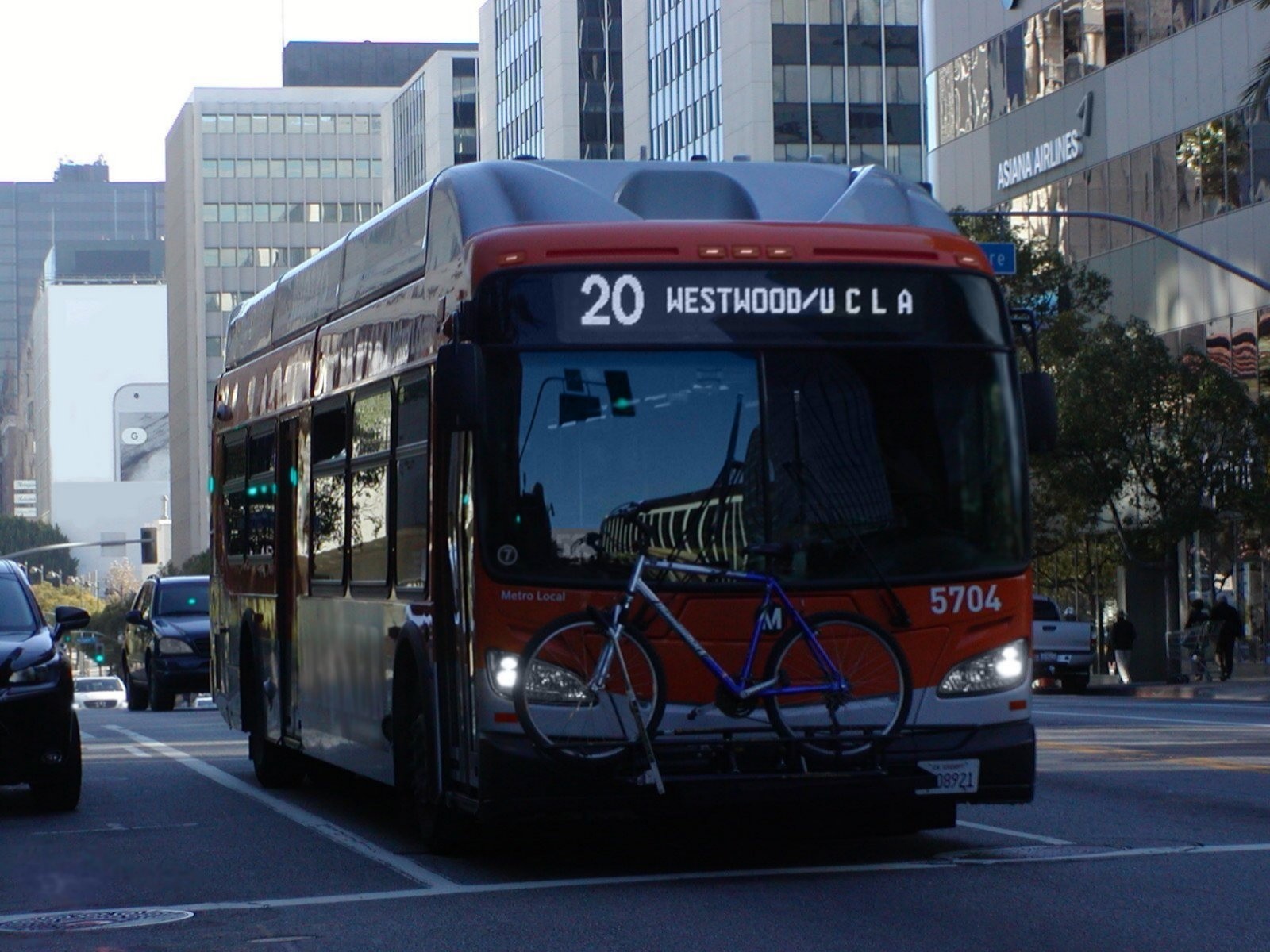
[509,497,914,775]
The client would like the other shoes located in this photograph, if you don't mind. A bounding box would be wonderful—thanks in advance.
[1220,671,1230,682]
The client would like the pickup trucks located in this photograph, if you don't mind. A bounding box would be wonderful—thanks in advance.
[1032,595,1097,690]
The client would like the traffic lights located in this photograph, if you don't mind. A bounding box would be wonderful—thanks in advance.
[603,370,637,418]
[95,643,105,664]
[63,628,72,644]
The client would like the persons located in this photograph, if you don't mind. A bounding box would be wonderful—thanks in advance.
[1063,607,1076,622]
[1110,609,1136,684]
[1185,595,1245,681]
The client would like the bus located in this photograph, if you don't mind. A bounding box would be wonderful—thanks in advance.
[582,481,751,584]
[204,155,1067,860]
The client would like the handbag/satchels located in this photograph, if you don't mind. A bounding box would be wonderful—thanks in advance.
[1183,632,1198,648]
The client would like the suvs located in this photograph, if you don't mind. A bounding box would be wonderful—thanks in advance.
[0,559,89,813]
[123,574,213,712]
[72,676,129,712]
